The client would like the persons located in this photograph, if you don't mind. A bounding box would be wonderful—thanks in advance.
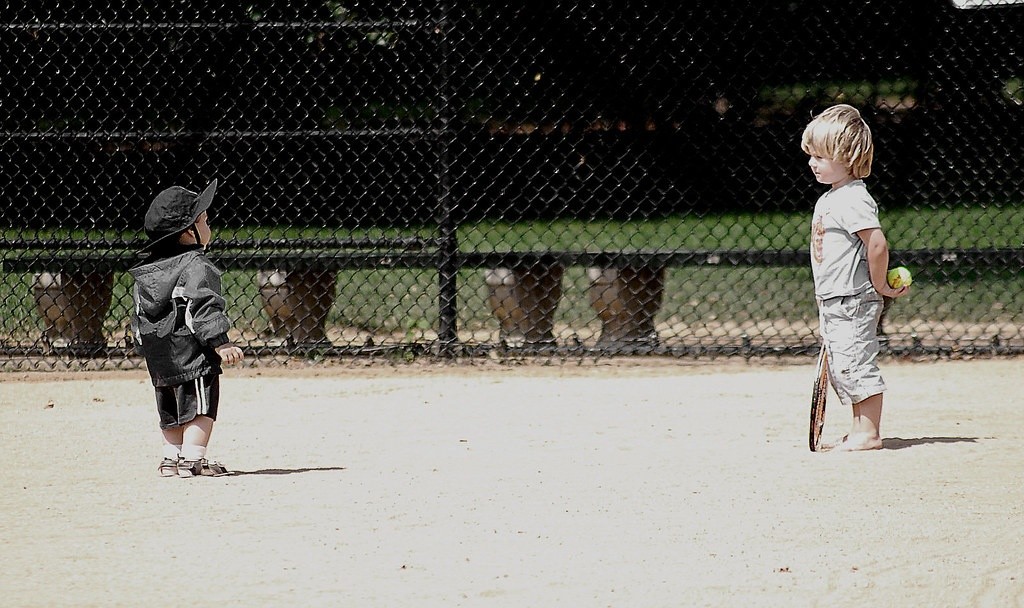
[127,178,243,479]
[800,103,910,453]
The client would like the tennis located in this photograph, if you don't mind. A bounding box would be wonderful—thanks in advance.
[888,266,912,289]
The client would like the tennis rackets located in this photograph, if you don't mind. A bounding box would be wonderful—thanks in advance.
[808,338,829,452]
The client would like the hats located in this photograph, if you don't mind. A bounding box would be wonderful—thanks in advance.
[136,179,218,255]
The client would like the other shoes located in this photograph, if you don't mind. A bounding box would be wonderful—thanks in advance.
[178,460,226,479]
[159,459,197,477]
